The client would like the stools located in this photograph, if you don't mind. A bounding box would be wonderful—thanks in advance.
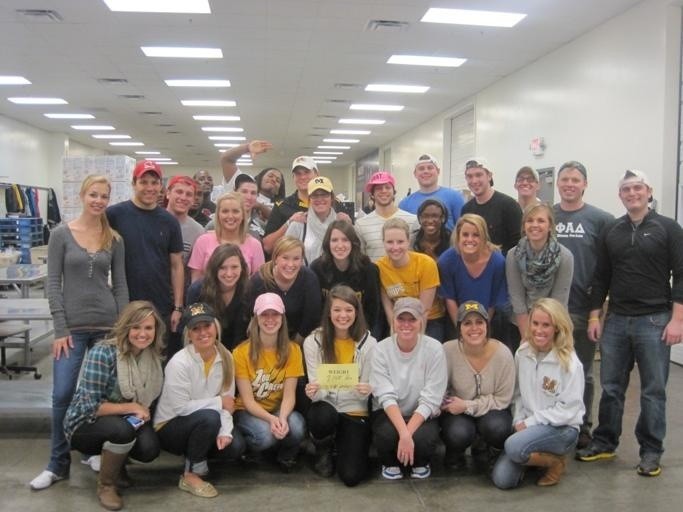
[1,324,34,379]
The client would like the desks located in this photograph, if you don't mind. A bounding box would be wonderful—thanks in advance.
[0,264,53,379]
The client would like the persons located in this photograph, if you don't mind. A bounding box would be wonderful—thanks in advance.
[153,302,236,499]
[233,172,264,239]
[231,292,307,474]
[369,296,448,481]
[238,236,323,344]
[308,219,382,341]
[408,198,454,262]
[62,299,165,509]
[302,283,378,487]
[491,297,587,490]
[549,160,617,452]
[440,214,508,342]
[188,192,266,284]
[219,139,285,229]
[443,299,516,474]
[459,157,524,257]
[372,218,447,346]
[187,169,218,227]
[285,176,339,267]
[355,172,422,266]
[397,153,466,232]
[103,159,185,370]
[29,173,130,491]
[514,164,546,220]
[264,156,353,255]
[575,167,683,476]
[165,173,206,268]
[506,206,575,354]
[176,242,248,351]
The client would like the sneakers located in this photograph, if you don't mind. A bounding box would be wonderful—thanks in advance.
[576,447,617,461]
[576,428,594,449]
[79,454,101,472]
[383,465,404,479]
[410,464,431,479]
[30,470,66,490]
[636,451,663,476]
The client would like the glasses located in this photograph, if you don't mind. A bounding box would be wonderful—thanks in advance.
[517,175,536,183]
[310,194,330,199]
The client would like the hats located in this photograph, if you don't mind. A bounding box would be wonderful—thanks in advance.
[179,303,218,331]
[456,299,489,323]
[307,177,335,196]
[292,156,319,172]
[234,173,256,191]
[465,157,494,176]
[412,153,441,176]
[131,160,163,179]
[253,293,286,317]
[558,160,588,179]
[167,175,198,191]
[514,165,540,187]
[393,296,426,322]
[364,171,395,192]
[619,168,653,189]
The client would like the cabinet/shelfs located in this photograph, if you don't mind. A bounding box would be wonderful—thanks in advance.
[0,218,46,264]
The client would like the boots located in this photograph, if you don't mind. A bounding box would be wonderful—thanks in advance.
[522,450,564,486]
[311,436,336,477]
[99,436,136,510]
[118,466,137,487]
[486,444,505,478]
[283,445,306,471]
[245,449,265,471]
[444,446,466,470]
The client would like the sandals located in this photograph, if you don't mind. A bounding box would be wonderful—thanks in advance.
[179,475,218,498]
